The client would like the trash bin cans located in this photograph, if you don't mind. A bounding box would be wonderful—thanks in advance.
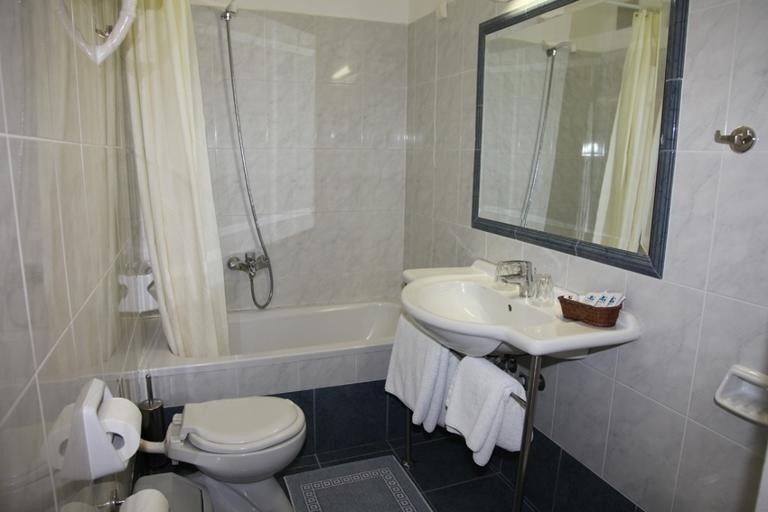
[132,472,213,512]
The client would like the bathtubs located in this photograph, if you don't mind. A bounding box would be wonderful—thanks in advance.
[135,301,401,409]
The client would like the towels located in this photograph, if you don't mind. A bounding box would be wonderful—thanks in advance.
[444,357,534,466]
[385,316,462,431]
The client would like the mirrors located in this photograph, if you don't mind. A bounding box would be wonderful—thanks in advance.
[470,0,690,279]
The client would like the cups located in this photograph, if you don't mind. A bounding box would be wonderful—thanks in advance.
[528,273,556,308]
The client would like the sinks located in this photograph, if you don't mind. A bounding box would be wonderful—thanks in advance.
[400,258,643,358]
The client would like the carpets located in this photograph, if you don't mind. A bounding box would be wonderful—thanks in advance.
[284,453,434,512]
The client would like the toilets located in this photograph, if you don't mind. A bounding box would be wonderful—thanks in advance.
[165,395,307,511]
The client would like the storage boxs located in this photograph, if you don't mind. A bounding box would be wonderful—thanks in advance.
[559,294,622,327]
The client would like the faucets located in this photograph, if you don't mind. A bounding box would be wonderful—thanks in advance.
[495,260,536,297]
